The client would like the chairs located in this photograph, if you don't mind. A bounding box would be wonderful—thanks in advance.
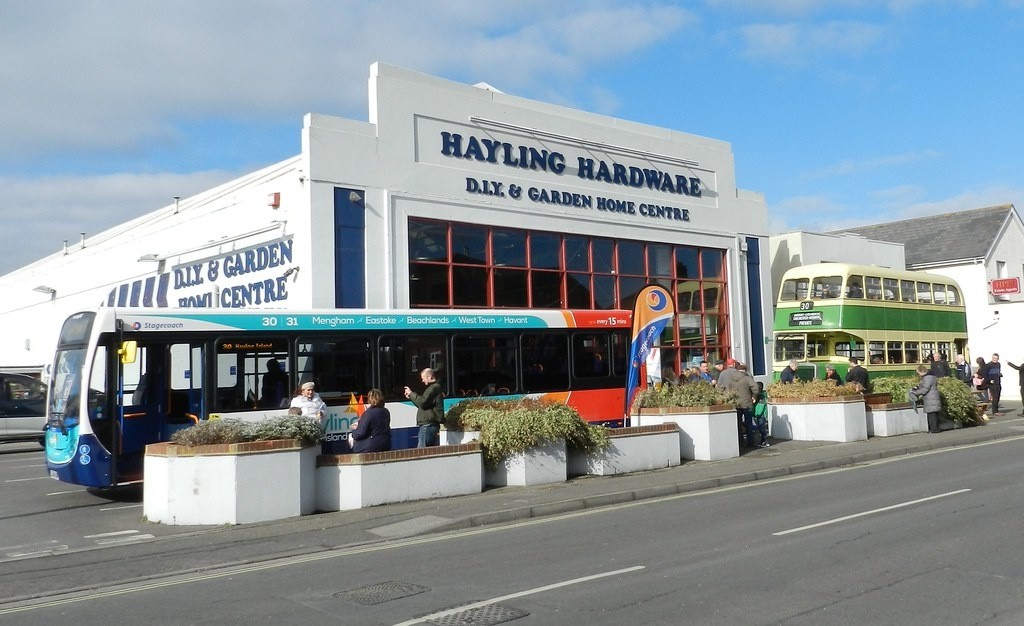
[867,295,943,304]
[460,387,510,397]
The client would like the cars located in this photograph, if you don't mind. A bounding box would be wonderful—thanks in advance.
[0,366,106,446]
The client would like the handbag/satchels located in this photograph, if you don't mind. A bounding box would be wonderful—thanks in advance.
[972,377,983,385]
[346,432,355,449]
[908,385,923,410]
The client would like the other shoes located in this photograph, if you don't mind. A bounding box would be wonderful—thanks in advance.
[757,441,770,447]
[993,412,1005,416]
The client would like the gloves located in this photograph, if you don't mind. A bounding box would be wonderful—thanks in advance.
[754,418,759,424]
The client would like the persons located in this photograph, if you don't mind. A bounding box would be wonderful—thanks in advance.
[257,359,290,408]
[404,368,444,448]
[349,389,392,453]
[822,282,894,301]
[677,353,1024,447]
[289,381,327,456]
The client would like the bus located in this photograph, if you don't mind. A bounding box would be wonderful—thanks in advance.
[655,276,727,394]
[772,262,970,403]
[41,307,635,497]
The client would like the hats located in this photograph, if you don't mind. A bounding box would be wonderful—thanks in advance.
[716,360,724,364]
[301,382,315,389]
[727,359,737,365]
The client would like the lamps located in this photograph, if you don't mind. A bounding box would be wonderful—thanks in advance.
[137,254,165,262]
[34,286,56,293]
